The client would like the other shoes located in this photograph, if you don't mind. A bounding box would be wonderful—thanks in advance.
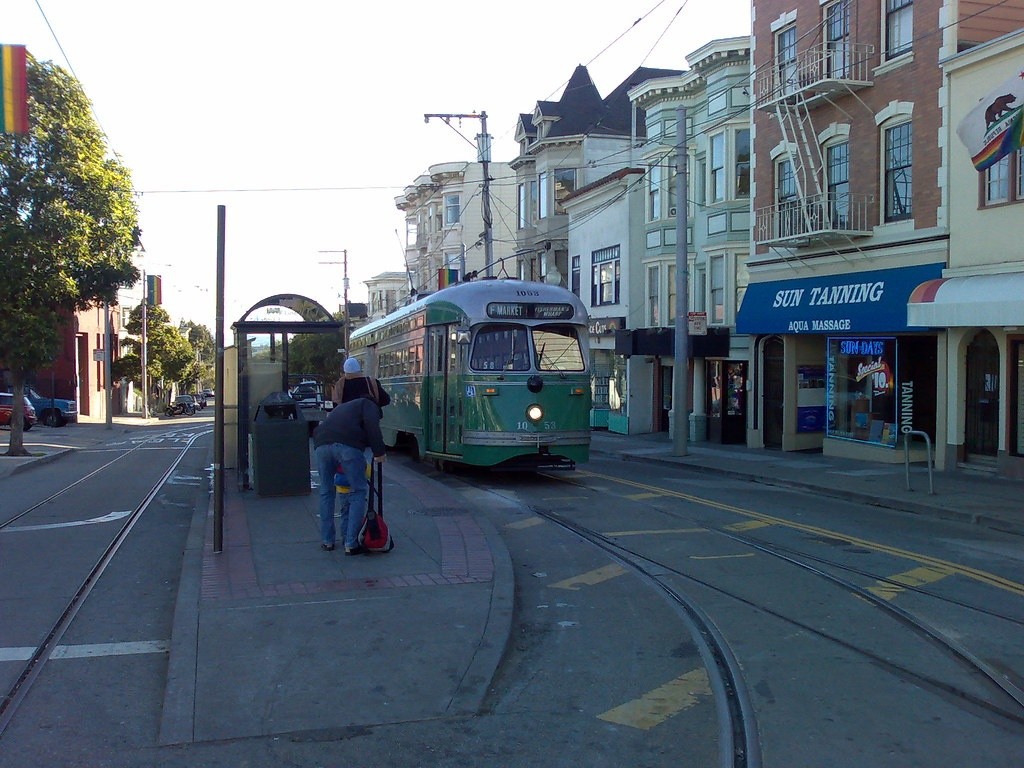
[344,546,369,556]
[320,543,335,551]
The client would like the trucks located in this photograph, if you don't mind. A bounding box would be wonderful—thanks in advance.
[289,382,321,408]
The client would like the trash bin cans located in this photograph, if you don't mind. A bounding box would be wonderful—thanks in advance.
[252,391,312,498]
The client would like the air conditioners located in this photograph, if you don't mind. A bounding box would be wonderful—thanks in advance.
[669,208,677,216]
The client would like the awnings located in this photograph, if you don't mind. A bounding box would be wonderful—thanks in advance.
[735,261,946,334]
[906,272,1024,326]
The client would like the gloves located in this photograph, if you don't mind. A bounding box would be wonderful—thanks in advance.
[374,454,388,463]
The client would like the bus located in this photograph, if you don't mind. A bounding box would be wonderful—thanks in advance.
[348,273,595,476]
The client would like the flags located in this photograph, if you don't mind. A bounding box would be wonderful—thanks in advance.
[956,65,1024,173]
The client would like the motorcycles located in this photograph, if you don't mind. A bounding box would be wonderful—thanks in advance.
[164,402,194,417]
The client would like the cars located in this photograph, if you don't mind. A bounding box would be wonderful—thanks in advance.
[203,389,211,397]
[175,394,206,411]
[0,393,38,433]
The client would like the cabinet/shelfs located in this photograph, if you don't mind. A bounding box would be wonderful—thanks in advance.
[592,363,627,413]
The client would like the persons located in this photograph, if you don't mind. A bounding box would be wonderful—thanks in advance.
[312,395,386,556]
[334,460,371,545]
[332,358,390,407]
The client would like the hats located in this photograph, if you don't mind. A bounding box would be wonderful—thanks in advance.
[343,358,361,373]
[360,394,384,420]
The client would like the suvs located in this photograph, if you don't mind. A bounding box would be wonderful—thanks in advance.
[8,387,78,428]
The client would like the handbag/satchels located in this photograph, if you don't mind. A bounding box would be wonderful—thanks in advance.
[376,379,390,406]
[358,510,394,553]
